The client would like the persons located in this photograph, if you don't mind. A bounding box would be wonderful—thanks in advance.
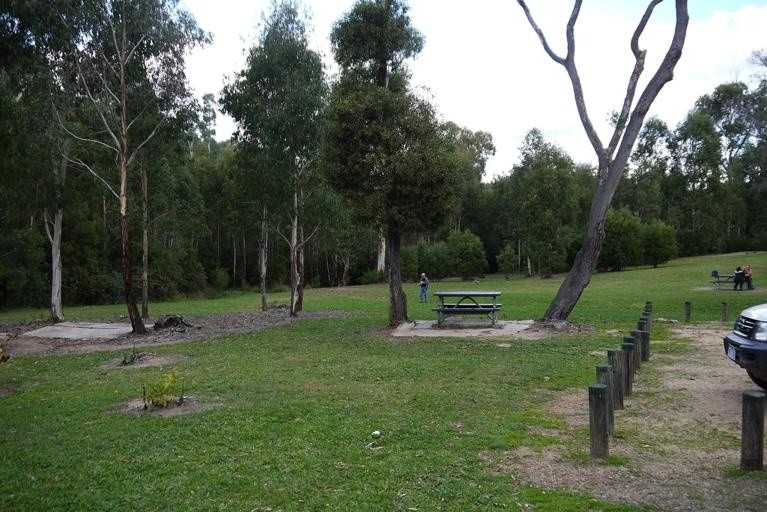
[734,266,746,290]
[417,273,430,303]
[743,265,754,290]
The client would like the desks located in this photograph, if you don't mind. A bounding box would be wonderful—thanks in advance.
[714,274,748,290]
[433,292,502,327]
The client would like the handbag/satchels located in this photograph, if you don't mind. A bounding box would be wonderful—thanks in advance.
[712,271,718,276]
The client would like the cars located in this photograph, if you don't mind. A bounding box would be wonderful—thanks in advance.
[724,302,767,391]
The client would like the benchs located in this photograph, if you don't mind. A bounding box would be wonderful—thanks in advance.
[709,280,747,283]
[438,302,502,307]
[432,308,501,312]
